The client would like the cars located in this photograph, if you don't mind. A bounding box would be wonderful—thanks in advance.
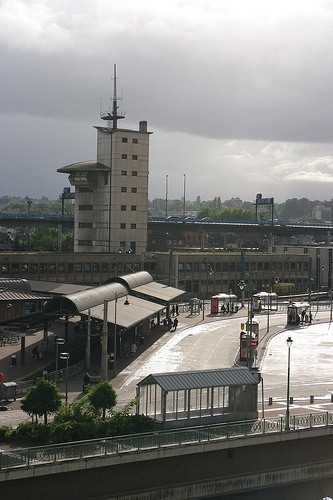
[200,216,213,222]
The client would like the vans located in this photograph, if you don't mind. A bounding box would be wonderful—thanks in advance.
[184,217,195,222]
[166,216,178,222]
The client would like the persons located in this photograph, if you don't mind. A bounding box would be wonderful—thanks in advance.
[301,310,305,321]
[297,314,300,321]
[221,305,225,310]
[84,373,90,385]
[227,303,229,312]
[200,302,202,310]
[172,304,178,316]
[174,318,178,330]
[32,345,39,356]
[168,316,173,329]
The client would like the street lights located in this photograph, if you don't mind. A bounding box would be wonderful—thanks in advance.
[203,264,214,321]
[236,272,252,367]
[60,353,70,405]
[284,336,292,430]
[247,289,262,367]
[113,292,130,377]
[165,174,168,219]
[182,173,187,223]
[267,271,280,331]
[54,338,66,383]
